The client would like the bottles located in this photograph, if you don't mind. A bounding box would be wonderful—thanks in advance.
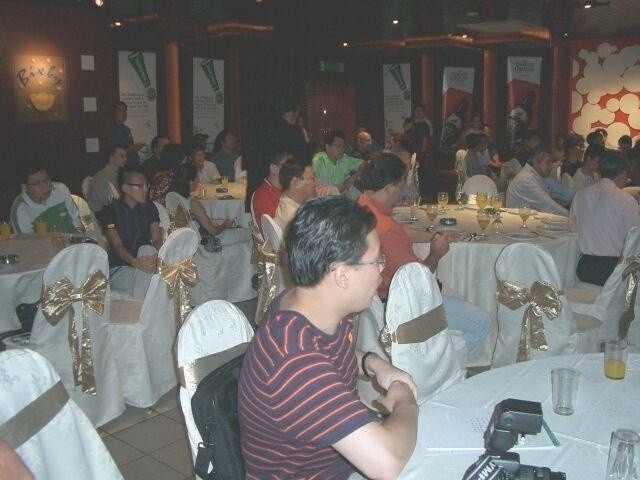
[128,51,150,88]
[506,89,535,138]
[439,98,470,146]
[386,63,409,92]
[201,58,220,91]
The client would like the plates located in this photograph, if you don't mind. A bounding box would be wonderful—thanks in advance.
[536,224,569,233]
[504,232,535,242]
[465,205,489,210]
[509,209,537,216]
[542,216,568,225]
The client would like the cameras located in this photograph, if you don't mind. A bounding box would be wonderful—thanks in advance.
[461,399,566,480]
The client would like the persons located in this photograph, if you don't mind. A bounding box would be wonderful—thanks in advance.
[353,152,495,361]
[230,193,424,479]
[507,104,640,287]
[246,99,511,253]
[0,439,38,480]
[84,101,247,302]
[9,164,109,245]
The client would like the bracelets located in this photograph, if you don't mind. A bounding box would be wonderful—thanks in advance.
[361,349,377,380]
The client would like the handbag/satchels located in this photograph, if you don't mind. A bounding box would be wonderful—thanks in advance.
[190,353,246,480]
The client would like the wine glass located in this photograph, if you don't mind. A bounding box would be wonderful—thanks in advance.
[476,191,488,219]
[425,203,440,231]
[477,210,492,238]
[491,209,506,240]
[517,203,531,231]
[436,190,449,215]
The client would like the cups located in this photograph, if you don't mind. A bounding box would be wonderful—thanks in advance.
[409,200,420,221]
[73,225,86,244]
[222,175,229,187]
[490,194,502,212]
[550,366,580,418]
[36,219,48,238]
[52,235,65,256]
[200,186,207,199]
[605,428,640,480]
[0,222,11,239]
[603,335,629,382]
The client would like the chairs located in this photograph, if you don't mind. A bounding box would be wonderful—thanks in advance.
[0,150,640,480]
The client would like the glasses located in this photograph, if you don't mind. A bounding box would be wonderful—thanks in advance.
[332,253,386,272]
